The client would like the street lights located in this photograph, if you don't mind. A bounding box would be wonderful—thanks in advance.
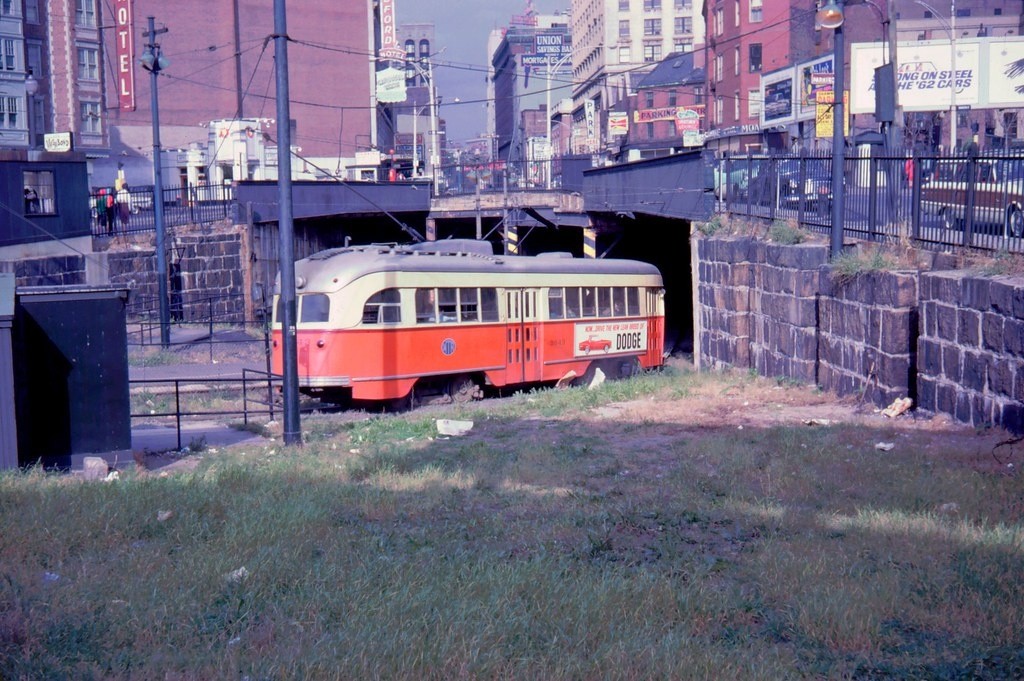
[24,74,39,151]
[369,56,439,195]
[413,98,460,179]
[139,41,170,353]
[546,45,617,188]
[815,2,845,261]
[537,119,575,153]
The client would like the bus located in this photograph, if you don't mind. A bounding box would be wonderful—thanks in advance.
[269,239,667,410]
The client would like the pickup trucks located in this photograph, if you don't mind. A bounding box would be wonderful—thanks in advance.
[89,185,154,218]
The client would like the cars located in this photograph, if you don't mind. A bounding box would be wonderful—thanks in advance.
[752,154,846,212]
[919,155,1024,240]
[712,155,769,199]
